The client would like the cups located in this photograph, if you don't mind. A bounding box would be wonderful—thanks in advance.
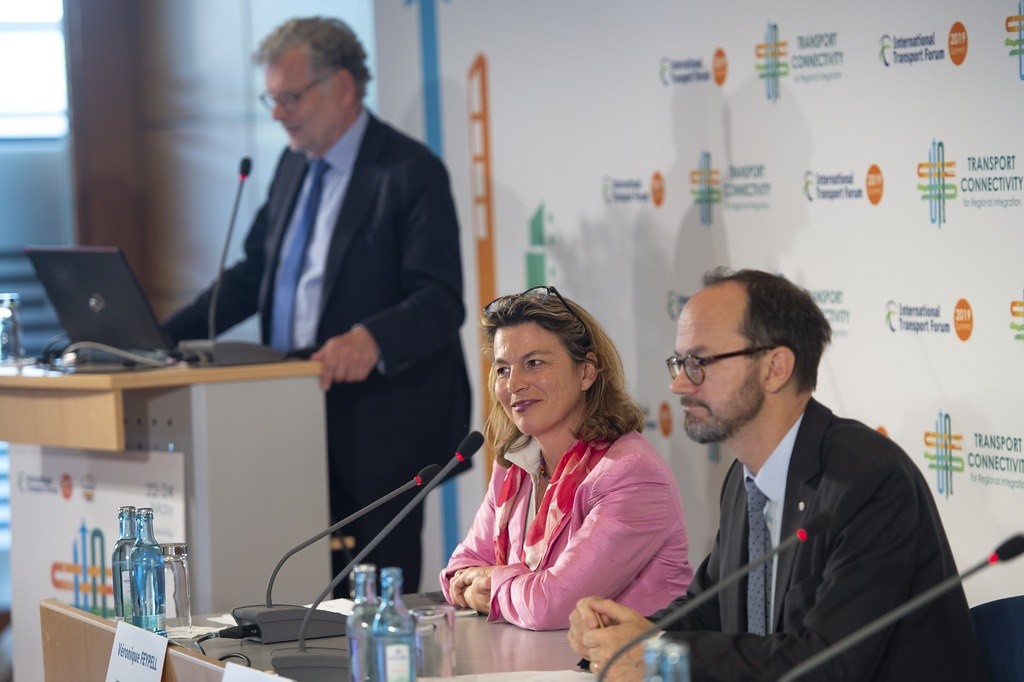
[409,605,455,677]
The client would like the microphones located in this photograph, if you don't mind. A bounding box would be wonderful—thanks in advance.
[270,431,485,682]
[597,511,833,682]
[230,463,441,644]
[777,534,1024,682]
[175,157,300,368]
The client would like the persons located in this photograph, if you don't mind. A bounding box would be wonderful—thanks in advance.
[162,17,472,597]
[439,285,693,631]
[568,266,984,682]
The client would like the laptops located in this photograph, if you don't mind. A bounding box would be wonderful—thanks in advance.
[25,246,178,366]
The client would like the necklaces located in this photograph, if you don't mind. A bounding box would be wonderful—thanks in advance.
[539,449,550,482]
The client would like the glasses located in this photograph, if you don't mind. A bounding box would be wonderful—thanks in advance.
[482,285,587,331]
[259,68,336,112]
[666,343,773,386]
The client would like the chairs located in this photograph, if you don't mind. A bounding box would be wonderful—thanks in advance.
[970,594,1024,682]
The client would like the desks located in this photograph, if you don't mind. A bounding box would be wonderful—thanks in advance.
[42,593,592,682]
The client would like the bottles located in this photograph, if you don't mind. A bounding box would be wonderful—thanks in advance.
[159,542,192,636]
[129,508,167,635]
[371,567,416,682]
[348,565,384,682]
[641,638,691,681]
[113,506,138,623]
[1,294,23,375]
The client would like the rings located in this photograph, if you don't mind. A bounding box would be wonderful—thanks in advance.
[455,572,462,578]
[594,663,598,673]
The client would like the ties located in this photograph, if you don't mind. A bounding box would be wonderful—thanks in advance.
[746,479,774,636]
[269,156,332,356]
